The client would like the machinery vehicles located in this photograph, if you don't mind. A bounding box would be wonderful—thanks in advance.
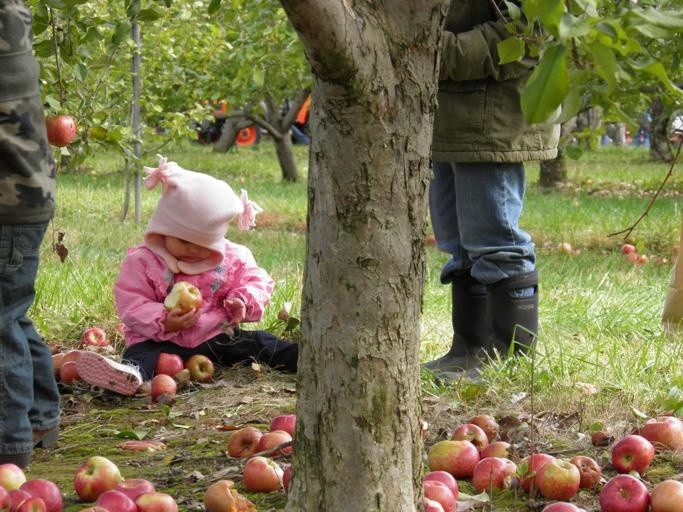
[167,79,310,148]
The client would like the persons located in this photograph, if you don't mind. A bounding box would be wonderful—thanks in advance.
[419,0,563,388]
[0,0,63,467]
[77,152,298,395]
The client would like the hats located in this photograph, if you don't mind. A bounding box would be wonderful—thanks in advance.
[142,153,263,276]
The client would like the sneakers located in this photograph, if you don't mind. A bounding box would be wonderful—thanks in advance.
[74,351,142,397]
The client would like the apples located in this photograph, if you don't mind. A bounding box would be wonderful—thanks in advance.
[45,115,77,148]
[540,235,682,265]
[417,414,682,512]
[164,282,203,316]
[424,236,437,247]
[0,327,296,512]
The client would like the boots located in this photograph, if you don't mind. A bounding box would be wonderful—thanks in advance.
[433,271,537,385]
[419,271,493,374]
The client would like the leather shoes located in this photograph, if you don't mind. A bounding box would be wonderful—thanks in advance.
[32,427,58,449]
[0,453,30,468]
